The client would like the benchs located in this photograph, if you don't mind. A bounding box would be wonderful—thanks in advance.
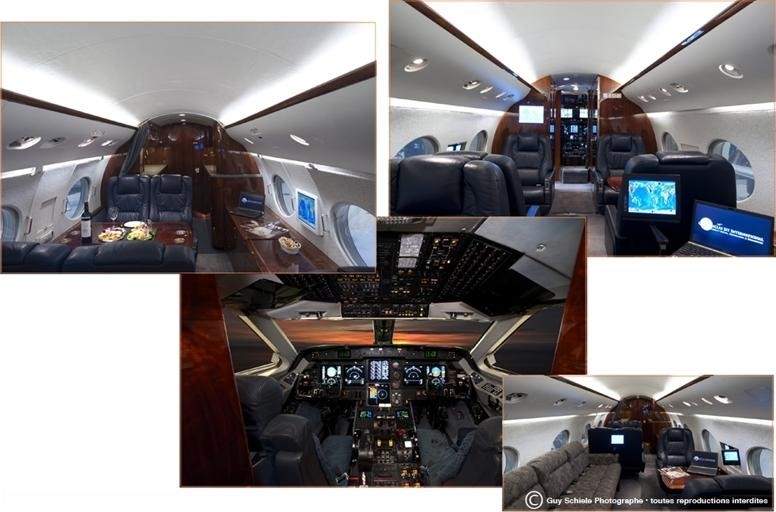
[585,426,647,481]
[606,419,642,428]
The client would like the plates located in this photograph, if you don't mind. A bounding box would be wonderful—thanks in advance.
[174,230,186,244]
[59,230,80,244]
[98,221,156,243]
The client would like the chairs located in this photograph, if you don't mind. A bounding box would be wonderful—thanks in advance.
[60,235,198,273]
[1,238,69,273]
[591,131,639,207]
[654,424,696,468]
[603,149,740,256]
[416,410,502,487]
[149,171,195,230]
[102,171,149,223]
[232,371,353,486]
[435,147,542,216]
[389,155,512,217]
[501,130,558,212]
[676,474,775,511]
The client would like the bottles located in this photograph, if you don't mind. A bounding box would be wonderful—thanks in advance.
[81,202,92,243]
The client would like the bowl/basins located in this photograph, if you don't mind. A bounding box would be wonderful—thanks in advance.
[278,237,301,255]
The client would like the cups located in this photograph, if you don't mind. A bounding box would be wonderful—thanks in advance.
[147,219,153,228]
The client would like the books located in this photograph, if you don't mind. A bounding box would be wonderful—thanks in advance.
[657,466,690,479]
[245,224,289,240]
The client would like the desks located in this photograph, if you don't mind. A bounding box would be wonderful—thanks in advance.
[603,173,621,194]
[654,464,728,496]
[54,215,190,245]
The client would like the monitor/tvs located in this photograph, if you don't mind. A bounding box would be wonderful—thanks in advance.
[722,450,741,465]
[622,173,681,222]
[518,104,545,123]
[295,188,323,236]
[611,435,624,444]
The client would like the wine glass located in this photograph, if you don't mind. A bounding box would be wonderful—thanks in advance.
[108,207,119,228]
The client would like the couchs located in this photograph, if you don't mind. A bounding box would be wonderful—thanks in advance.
[502,437,624,511]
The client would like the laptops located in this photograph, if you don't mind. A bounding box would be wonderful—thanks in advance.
[670,199,774,257]
[228,191,265,218]
[687,450,718,476]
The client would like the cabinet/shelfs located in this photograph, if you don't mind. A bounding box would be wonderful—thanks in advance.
[224,203,323,272]
